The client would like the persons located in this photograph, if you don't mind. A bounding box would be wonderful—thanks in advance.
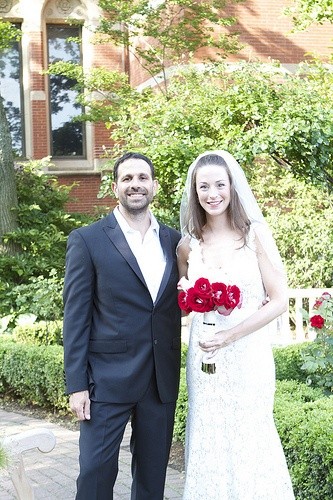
[62,152,270,500]
[175,149,297,500]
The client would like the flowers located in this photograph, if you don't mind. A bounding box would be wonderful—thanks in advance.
[177,277,244,373]
